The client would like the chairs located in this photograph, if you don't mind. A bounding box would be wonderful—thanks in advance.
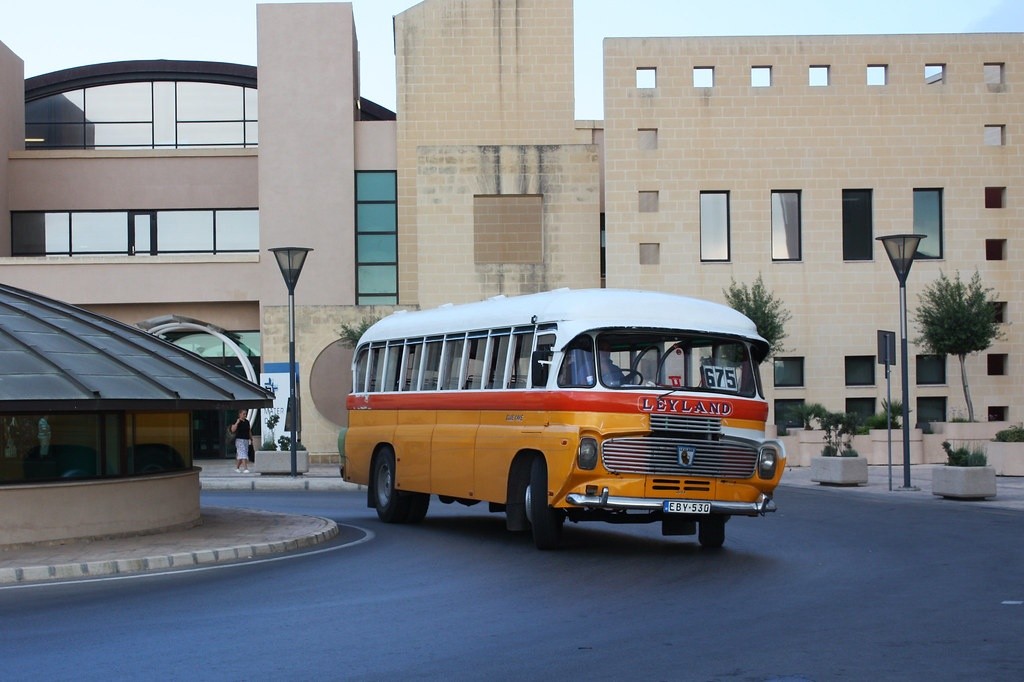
[367,375,528,393]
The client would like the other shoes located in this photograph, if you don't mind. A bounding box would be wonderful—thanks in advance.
[243,470,249,474]
[234,469,241,472]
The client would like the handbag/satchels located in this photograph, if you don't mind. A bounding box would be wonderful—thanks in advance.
[224,424,236,441]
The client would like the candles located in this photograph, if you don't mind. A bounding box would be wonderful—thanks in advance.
[933,440,997,496]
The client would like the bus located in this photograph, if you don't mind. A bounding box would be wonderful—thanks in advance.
[341,289,787,550]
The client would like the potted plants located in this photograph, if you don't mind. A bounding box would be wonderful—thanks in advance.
[254,414,309,473]
[990,422,1024,477]
[868,397,923,464]
[811,411,870,484]
[785,401,831,465]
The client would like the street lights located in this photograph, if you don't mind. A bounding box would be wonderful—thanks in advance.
[875,233,927,492]
[268,246,317,480]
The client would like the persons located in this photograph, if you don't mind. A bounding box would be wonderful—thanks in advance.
[599,352,630,384]
[231,410,251,473]
